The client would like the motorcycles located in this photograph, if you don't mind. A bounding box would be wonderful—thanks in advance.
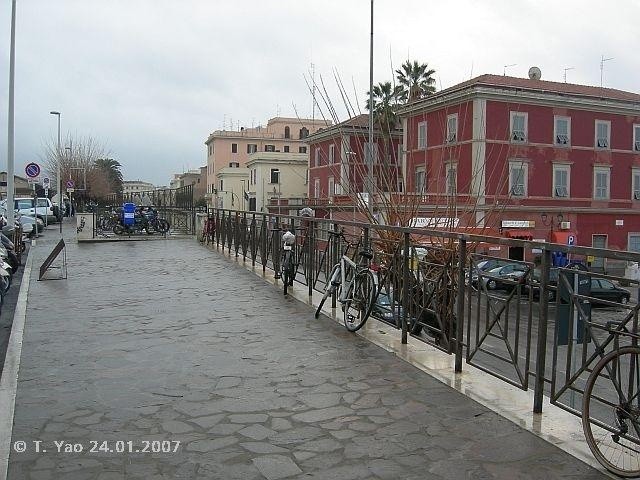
[112,206,171,236]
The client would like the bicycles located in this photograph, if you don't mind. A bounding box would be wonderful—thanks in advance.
[314,229,381,333]
[270,227,306,297]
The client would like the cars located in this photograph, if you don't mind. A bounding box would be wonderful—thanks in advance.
[0,197,57,238]
[590,277,632,306]
[371,287,423,334]
[469,260,558,301]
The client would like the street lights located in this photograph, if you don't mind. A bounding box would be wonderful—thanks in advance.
[541,210,564,243]
[65,147,72,217]
[274,170,281,214]
[50,111,61,210]
[346,151,357,235]
